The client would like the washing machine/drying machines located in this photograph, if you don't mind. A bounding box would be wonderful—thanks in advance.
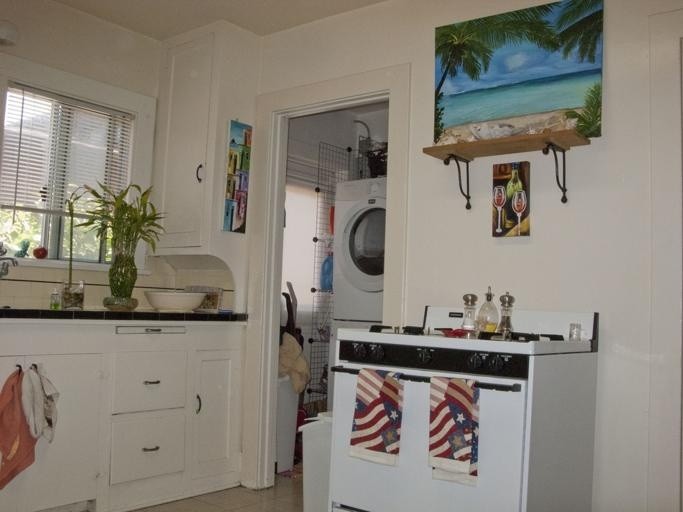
[332,177,386,322]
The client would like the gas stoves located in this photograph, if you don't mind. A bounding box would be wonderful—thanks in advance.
[336,326,594,378]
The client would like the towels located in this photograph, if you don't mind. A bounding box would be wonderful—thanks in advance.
[347,368,482,488]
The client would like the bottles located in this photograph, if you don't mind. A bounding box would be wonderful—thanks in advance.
[34,246,48,260]
[506,162,523,221]
[569,323,580,341]
[329,206,336,233]
[462,286,515,341]
[51,288,60,308]
[321,247,331,290]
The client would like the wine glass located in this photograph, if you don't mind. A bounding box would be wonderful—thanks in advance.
[493,185,527,236]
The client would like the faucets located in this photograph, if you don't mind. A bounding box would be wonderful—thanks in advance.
[0,257,18,267]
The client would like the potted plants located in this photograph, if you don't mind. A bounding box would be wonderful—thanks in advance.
[61,179,167,310]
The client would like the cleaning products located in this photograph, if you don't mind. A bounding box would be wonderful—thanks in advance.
[320,252,333,291]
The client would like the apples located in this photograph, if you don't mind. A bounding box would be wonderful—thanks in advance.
[34,247,47,258]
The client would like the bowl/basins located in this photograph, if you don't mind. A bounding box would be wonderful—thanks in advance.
[145,291,206,313]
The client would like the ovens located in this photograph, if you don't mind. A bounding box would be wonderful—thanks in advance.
[328,362,527,512]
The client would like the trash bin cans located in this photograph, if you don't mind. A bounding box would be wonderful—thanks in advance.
[298,412,333,512]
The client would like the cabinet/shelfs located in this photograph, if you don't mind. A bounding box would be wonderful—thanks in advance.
[148,18,256,258]
